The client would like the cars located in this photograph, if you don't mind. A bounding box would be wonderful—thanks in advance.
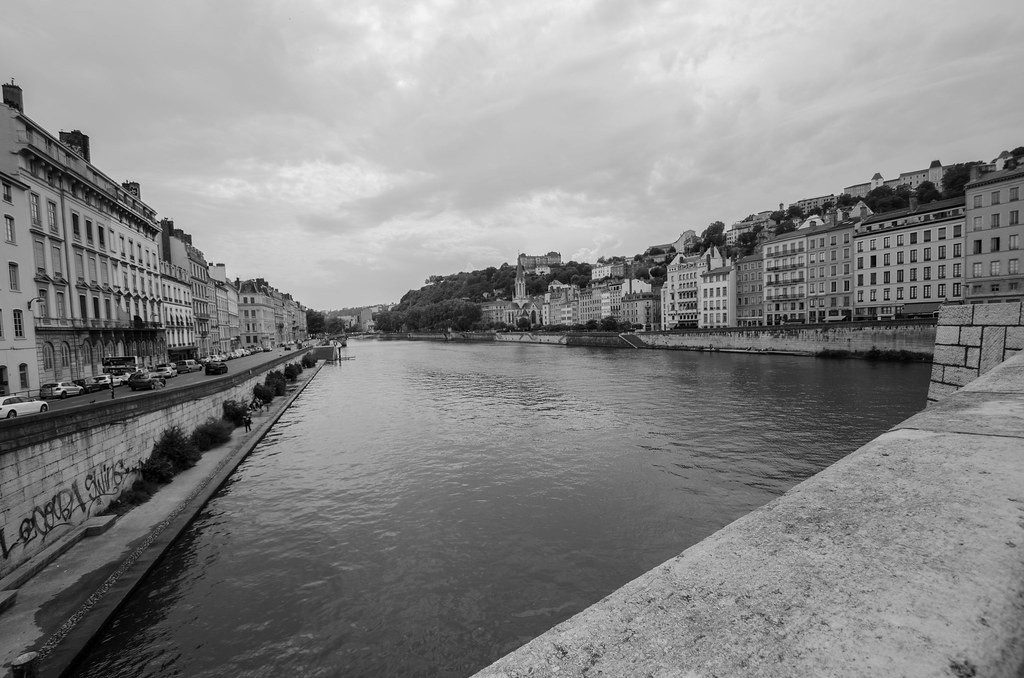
[157,367,179,378]
[205,362,228,375]
[199,345,272,364]
[128,372,167,391]
[39,381,85,400]
[96,374,124,389]
[157,364,177,370]
[0,395,50,420]
[115,371,130,383]
[74,377,104,393]
[285,345,291,350]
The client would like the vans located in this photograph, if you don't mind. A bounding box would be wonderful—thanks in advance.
[176,359,203,373]
